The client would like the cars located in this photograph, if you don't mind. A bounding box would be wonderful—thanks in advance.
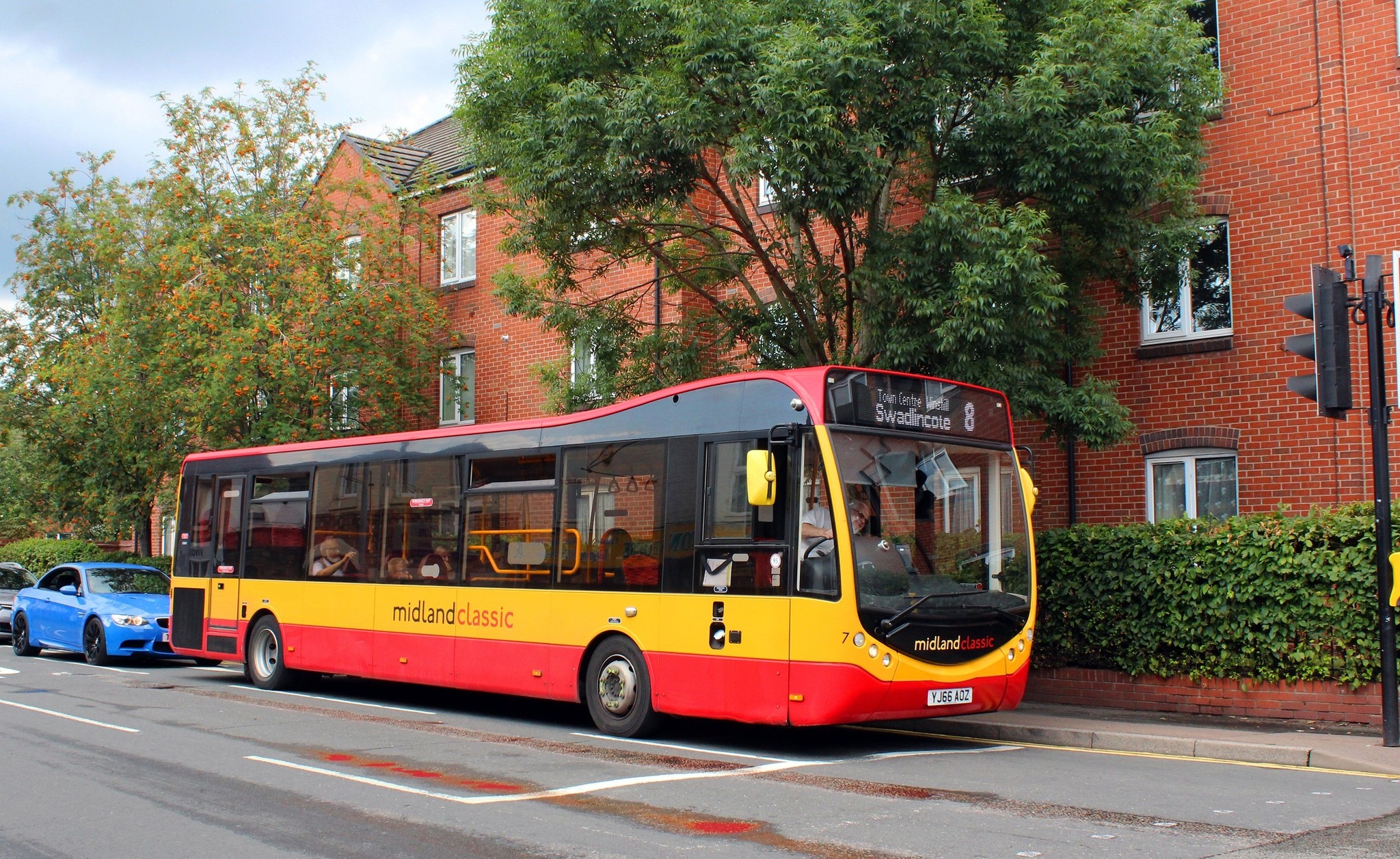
[0,562,40,646]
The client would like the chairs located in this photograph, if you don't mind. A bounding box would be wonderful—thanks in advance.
[198,508,486,582]
[597,527,633,589]
[623,553,659,586]
[56,573,77,590]
[116,571,137,592]
[747,536,784,587]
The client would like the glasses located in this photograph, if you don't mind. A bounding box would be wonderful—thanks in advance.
[395,569,406,573]
[850,507,869,526]
[326,547,341,551]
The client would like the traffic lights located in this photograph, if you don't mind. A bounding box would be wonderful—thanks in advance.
[1283,263,1353,422]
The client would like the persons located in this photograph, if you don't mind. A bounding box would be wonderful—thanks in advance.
[800,500,870,563]
[491,540,532,588]
[312,538,357,577]
[385,557,414,580]
[416,544,455,587]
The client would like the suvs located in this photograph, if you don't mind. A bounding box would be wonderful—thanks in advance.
[10,562,223,667]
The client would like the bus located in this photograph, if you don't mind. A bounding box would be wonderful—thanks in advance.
[161,362,1045,740]
